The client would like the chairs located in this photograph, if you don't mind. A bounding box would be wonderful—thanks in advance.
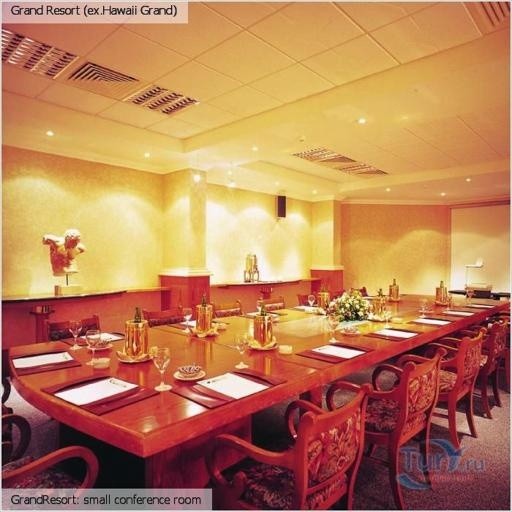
[2,414,100,510]
[45,287,510,511]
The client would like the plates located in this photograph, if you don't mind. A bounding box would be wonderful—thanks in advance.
[117,353,153,364]
[247,341,281,350]
[173,368,207,381]
[88,344,114,351]
[190,327,217,338]
[341,330,362,336]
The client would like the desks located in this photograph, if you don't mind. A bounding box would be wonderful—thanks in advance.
[7,293,510,509]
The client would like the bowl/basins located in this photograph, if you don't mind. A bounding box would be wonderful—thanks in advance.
[343,326,358,334]
[90,338,112,348]
[179,365,201,377]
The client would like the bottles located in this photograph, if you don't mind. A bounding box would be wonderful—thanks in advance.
[124,306,149,358]
[389,278,401,300]
[195,292,213,333]
[254,305,273,345]
[435,281,448,302]
[318,285,331,310]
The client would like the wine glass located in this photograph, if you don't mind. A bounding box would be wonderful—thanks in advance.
[382,311,393,328]
[182,308,193,333]
[326,316,340,343]
[150,348,173,391]
[68,319,84,350]
[234,335,250,369]
[418,298,429,318]
[85,330,100,365]
[465,288,475,304]
[308,294,316,313]
[255,300,265,319]
